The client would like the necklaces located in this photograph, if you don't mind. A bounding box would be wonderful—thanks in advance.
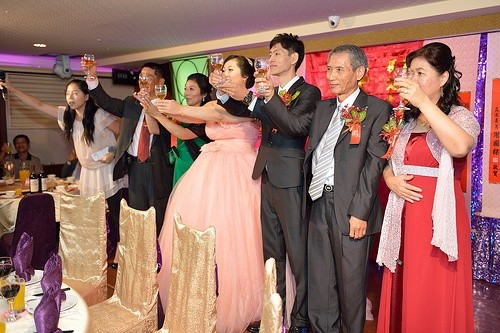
[416,116,430,126]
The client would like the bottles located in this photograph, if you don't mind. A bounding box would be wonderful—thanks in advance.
[29,160,40,193]
[38,161,48,192]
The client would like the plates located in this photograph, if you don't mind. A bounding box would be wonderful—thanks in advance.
[25,288,78,314]
[9,268,44,286]
[0,195,18,198]
[62,186,81,196]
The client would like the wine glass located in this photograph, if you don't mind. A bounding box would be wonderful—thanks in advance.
[139,73,152,99]
[0,275,20,322]
[154,85,167,114]
[83,53,96,80]
[392,68,413,111]
[210,53,225,86]
[254,58,269,94]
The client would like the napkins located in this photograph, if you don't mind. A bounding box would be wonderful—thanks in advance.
[33,252,64,333]
[11,229,34,282]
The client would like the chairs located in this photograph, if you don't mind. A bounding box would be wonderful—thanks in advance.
[0,189,283,333]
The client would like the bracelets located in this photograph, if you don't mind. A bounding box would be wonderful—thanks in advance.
[180,105,184,116]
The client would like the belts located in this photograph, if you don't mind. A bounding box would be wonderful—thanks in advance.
[324,184,334,192]
[129,155,150,164]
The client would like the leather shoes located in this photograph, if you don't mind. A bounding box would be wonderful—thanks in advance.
[288,324,309,333]
[246,319,261,333]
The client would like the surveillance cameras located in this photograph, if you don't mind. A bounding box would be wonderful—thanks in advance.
[328,16,340,28]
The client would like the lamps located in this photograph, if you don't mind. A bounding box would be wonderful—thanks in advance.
[51,54,72,78]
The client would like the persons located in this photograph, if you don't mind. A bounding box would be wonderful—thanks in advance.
[252,43,398,333]
[60,151,84,179]
[373,42,480,333]
[0,134,44,181]
[134,72,213,205]
[156,55,264,333]
[209,33,322,333]
[0,75,131,268]
[81,57,175,268]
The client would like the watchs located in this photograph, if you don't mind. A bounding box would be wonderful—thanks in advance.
[243,91,253,105]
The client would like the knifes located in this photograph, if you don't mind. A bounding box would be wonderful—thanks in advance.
[32,288,70,296]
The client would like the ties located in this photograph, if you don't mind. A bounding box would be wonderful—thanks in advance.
[136,115,150,163]
[307,104,344,202]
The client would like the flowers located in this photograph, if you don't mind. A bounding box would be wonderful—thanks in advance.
[272,89,300,134]
[378,114,406,160]
[338,103,368,144]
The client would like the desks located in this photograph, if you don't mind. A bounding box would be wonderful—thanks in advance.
[0,269,89,333]
[0,178,80,235]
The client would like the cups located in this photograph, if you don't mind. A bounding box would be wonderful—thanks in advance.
[6,191,16,197]
[15,182,22,196]
[46,174,75,192]
[0,276,9,304]
[0,316,7,333]
[5,143,13,154]
[4,162,30,185]
[11,278,25,314]
[0,257,13,275]
